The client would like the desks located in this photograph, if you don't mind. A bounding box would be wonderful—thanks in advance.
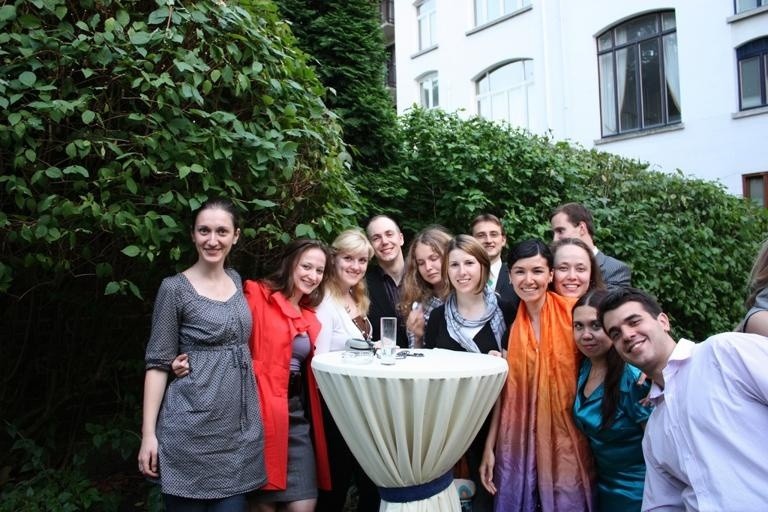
[309,346,508,512]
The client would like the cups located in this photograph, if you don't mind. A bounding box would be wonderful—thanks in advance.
[380,316,397,365]
